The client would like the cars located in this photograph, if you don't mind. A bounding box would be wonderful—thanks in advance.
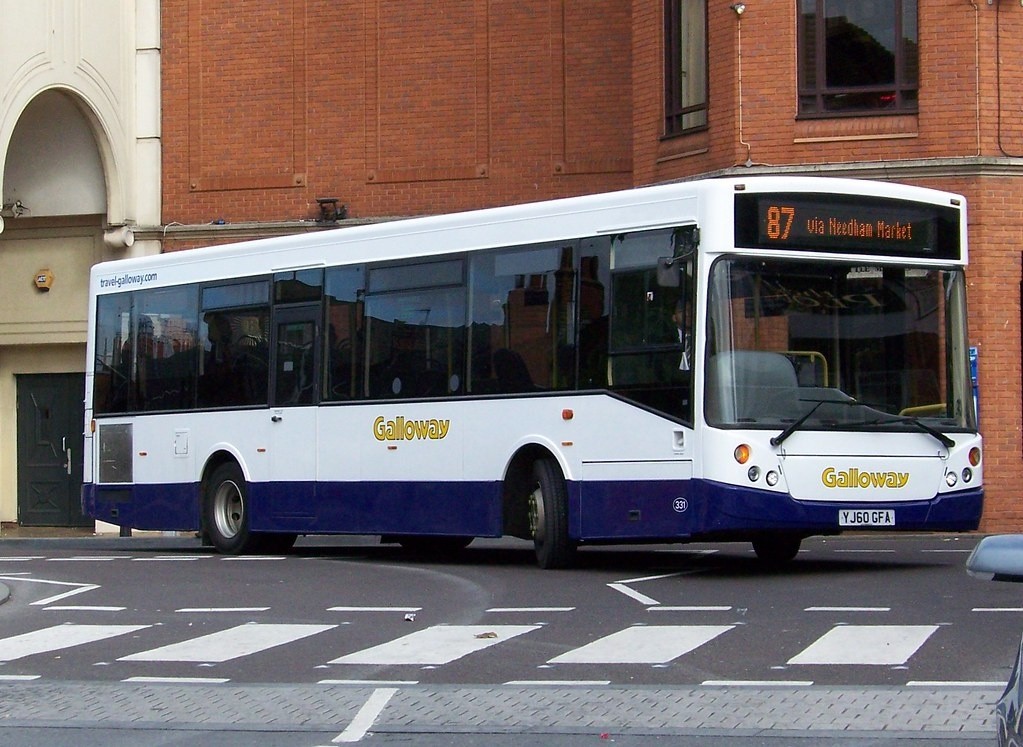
[964,532,1023,747]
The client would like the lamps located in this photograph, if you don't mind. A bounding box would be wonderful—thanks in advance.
[729,3,746,14]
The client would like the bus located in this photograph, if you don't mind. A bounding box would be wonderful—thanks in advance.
[79,176,984,572]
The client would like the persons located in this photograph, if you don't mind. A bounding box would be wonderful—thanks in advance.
[580,279,609,390]
[121,312,347,407]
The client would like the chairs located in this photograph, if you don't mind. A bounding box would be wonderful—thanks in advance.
[493,348,536,394]
[605,324,658,386]
